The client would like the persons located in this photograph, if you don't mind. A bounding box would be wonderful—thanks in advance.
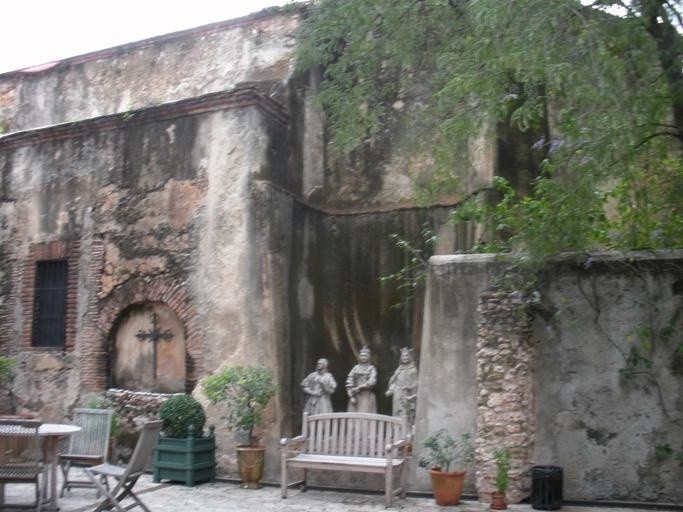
[298,358,338,415]
[343,346,377,413]
[384,347,417,423]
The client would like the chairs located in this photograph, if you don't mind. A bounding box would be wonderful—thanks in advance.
[56,406,110,500]
[0,419,50,512]
[89,418,168,512]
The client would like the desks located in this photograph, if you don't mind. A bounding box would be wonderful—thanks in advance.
[1,423,83,512]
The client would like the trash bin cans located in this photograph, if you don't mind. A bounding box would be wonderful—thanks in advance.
[531,465,564,511]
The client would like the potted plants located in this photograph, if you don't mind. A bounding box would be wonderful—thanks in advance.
[487,447,512,510]
[152,391,218,487]
[199,363,281,493]
[416,427,481,508]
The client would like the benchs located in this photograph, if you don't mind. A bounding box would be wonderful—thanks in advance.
[278,410,414,510]
